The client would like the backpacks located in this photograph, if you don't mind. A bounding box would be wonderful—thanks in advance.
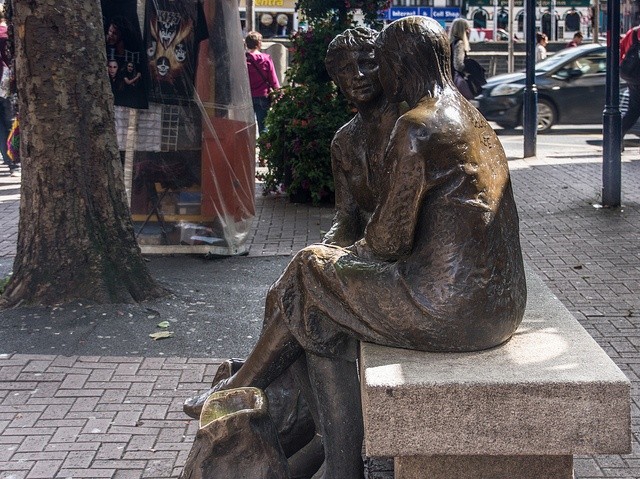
[620,27,640,82]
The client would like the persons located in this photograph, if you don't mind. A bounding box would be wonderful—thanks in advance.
[114,62,141,107]
[212,25,412,478]
[105,21,126,61]
[259,13,274,36]
[183,15,527,478]
[276,13,292,35]
[108,59,120,105]
[448,17,487,102]
[535,32,549,64]
[304,26,314,38]
[566,32,583,48]
[0,36,19,171]
[243,30,280,167]
[294,26,304,38]
[619,26,640,151]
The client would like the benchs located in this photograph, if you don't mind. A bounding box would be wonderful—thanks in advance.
[358,252,630,479]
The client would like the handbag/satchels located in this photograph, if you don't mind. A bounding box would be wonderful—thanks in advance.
[454,70,483,100]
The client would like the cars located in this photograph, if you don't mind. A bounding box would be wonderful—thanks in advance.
[469,42,639,134]
